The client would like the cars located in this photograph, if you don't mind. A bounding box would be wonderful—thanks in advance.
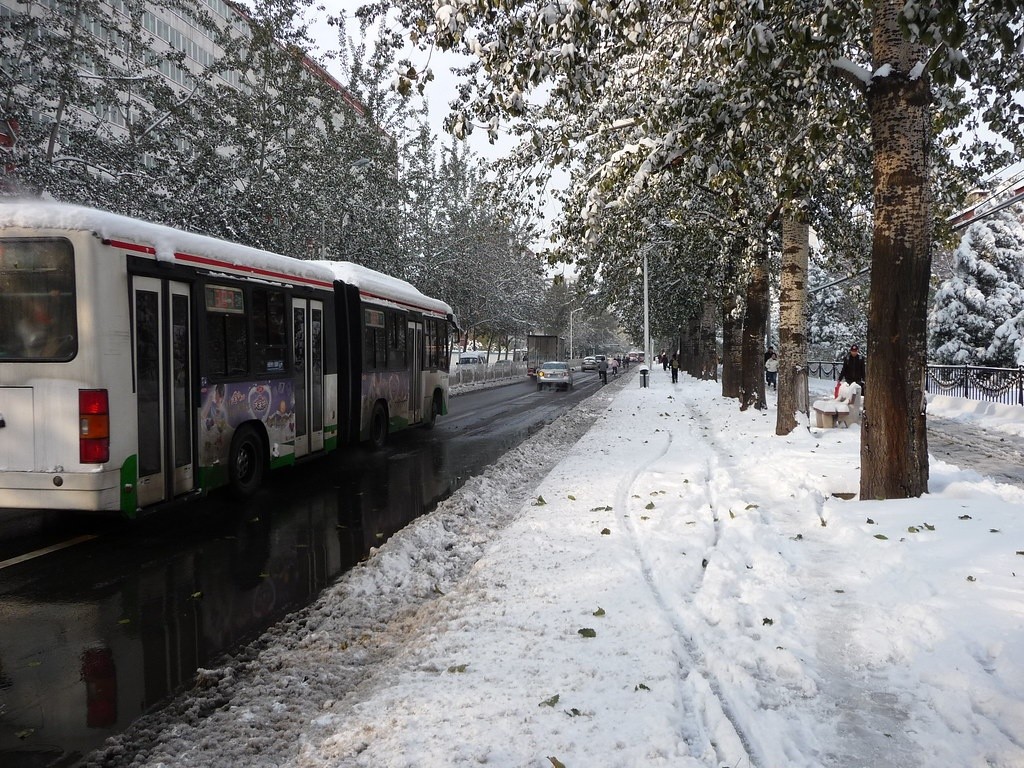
[492,359,514,373]
[580,356,598,372]
[536,361,575,391]
[607,352,645,368]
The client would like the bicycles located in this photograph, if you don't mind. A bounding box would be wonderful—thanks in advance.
[599,374,606,387]
[613,365,618,377]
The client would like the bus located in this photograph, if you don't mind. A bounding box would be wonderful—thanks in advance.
[0,206,469,519]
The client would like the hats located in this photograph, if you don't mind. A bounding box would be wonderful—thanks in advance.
[851,345,858,352]
[601,357,605,360]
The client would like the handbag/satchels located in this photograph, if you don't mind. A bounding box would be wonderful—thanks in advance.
[861,380,865,396]
[835,383,842,398]
[673,359,678,369]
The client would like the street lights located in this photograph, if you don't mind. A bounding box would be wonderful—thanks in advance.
[570,307,583,359]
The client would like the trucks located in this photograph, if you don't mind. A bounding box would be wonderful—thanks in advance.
[525,334,566,373]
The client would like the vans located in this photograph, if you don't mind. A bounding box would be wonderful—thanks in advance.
[455,351,486,377]
[595,354,608,368]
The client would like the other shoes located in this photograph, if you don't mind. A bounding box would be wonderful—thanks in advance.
[774,389,777,391]
[599,378,601,379]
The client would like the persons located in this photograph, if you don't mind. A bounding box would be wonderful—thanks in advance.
[617,356,621,367]
[764,346,779,391]
[523,356,526,361]
[598,358,608,384]
[662,354,668,371]
[837,345,865,396]
[621,356,630,369]
[611,358,619,374]
[671,354,681,383]
[202,384,227,422]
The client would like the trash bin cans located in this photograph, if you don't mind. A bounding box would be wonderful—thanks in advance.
[640,368,649,388]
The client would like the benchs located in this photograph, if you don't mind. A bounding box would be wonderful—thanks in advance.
[813,382,861,429]
